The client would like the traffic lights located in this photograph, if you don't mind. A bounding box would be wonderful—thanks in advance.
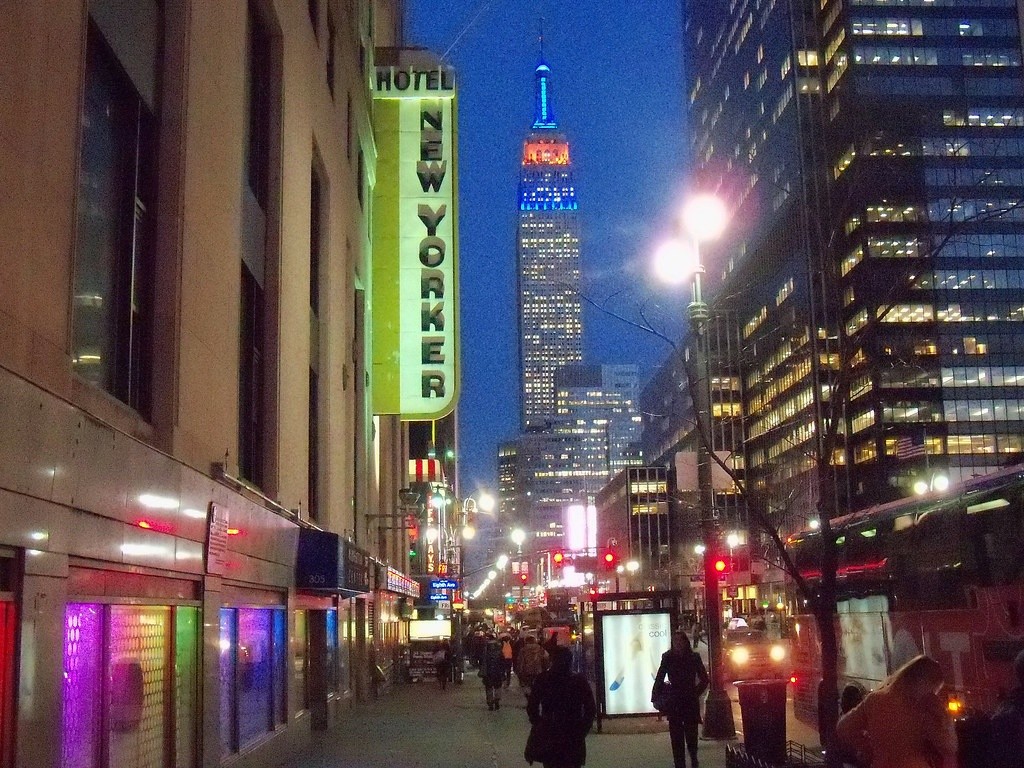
[603,552,615,566]
[552,550,564,567]
[520,573,527,582]
[709,557,730,573]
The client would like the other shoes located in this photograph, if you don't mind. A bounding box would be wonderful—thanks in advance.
[488,704,494,711]
[495,702,500,710]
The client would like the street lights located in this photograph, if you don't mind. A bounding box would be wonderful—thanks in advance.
[651,193,735,740]
[512,530,526,604]
[497,554,509,617]
[914,470,949,494]
[432,485,495,650]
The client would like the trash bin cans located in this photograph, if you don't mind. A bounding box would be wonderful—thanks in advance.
[733,679,787,762]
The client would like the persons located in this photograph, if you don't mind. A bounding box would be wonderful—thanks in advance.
[836,650,960,768]
[649,632,711,768]
[990,649,1023,767]
[462,620,597,768]
[690,620,708,650]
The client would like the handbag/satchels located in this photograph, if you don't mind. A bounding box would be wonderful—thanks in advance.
[478,658,488,677]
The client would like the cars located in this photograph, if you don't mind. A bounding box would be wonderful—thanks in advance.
[720,629,787,680]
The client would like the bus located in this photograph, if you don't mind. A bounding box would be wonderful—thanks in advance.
[790,463,1024,768]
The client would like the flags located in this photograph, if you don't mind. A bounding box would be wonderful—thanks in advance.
[896,431,926,460]
